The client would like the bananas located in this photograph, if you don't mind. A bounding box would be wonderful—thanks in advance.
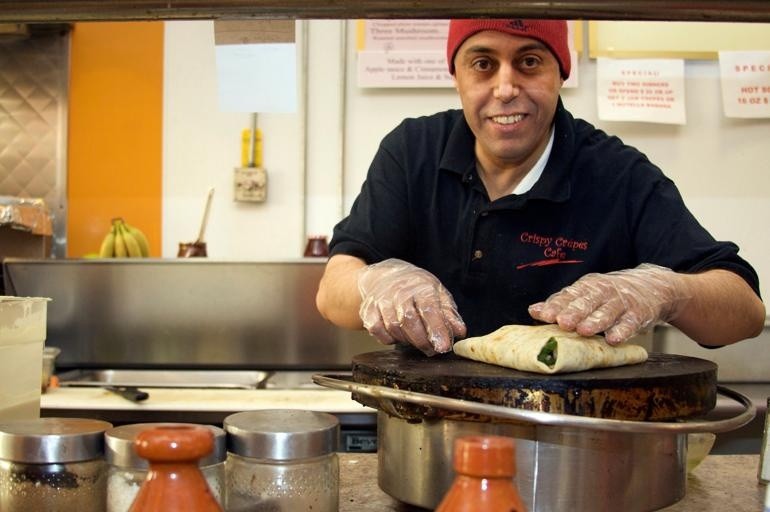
[99,216,149,258]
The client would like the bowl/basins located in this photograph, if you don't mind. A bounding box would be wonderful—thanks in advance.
[686,433,717,476]
[41,346,62,393]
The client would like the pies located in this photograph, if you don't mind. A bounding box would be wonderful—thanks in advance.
[453,323,649,373]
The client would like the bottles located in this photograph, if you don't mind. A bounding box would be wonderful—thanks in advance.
[435,435,529,512]
[222,410,341,512]
[0,410,112,512]
[757,397,770,485]
[304,236,329,257]
[126,423,224,512]
[104,411,227,512]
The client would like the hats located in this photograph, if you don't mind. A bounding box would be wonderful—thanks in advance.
[447,19,570,80]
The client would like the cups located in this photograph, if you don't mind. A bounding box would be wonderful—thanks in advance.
[0,296,52,422]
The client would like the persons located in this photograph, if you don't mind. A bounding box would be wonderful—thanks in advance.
[314,17,766,358]
